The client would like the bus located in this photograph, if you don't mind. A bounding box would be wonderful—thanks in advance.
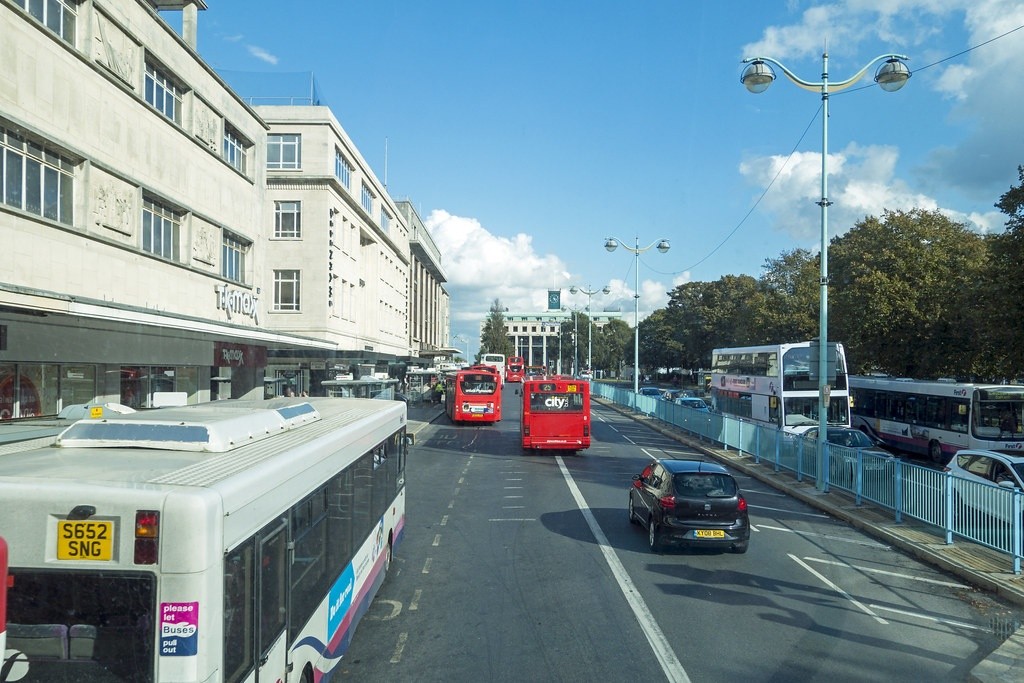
[507,356,525,383]
[480,353,506,389]
[0,395,414,682]
[462,364,498,383]
[444,370,501,424]
[515,375,591,450]
[847,375,1024,464]
[711,341,856,454]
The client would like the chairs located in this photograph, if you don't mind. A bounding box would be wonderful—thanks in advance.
[6,622,147,661]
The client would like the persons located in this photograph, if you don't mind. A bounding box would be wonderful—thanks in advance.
[286,386,309,397]
[435,380,446,404]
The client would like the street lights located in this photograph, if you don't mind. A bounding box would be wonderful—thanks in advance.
[561,303,590,380]
[520,338,523,357]
[461,338,468,361]
[603,236,671,394]
[569,284,610,380]
[740,37,912,486]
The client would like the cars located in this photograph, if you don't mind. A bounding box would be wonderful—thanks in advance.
[942,448,1024,530]
[662,390,690,404]
[579,370,593,379]
[799,425,899,480]
[628,458,750,553]
[793,426,896,481]
[676,397,711,426]
[638,387,662,403]
